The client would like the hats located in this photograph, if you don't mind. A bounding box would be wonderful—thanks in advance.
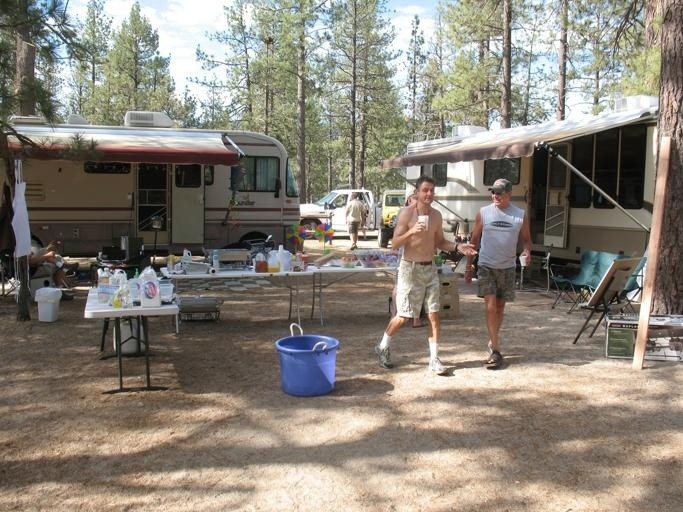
[488,178,513,194]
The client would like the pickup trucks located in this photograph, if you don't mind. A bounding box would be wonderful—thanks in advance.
[299,187,382,240]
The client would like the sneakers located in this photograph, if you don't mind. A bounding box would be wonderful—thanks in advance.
[61,263,80,275]
[375,345,394,369]
[351,243,355,250]
[356,246,358,249]
[428,358,448,375]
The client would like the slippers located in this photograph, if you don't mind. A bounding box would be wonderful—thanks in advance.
[485,341,493,353]
[486,351,502,369]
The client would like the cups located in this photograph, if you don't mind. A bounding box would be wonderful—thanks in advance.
[419,216,429,231]
[519,256,526,266]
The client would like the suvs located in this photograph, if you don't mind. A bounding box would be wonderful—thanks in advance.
[378,190,408,248]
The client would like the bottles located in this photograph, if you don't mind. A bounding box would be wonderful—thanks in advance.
[212,250,219,273]
[135,268,139,278]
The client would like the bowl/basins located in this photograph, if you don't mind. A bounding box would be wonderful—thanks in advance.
[355,251,383,267]
[333,252,357,268]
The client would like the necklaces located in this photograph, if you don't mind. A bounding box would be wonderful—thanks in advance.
[415,202,428,216]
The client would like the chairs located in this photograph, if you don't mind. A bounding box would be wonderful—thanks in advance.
[572,266,633,344]
[521,246,550,292]
[550,249,647,313]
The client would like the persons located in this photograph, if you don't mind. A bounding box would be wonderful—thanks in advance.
[465,179,531,367]
[375,177,477,375]
[390,192,429,328]
[346,192,365,250]
[22,251,79,300]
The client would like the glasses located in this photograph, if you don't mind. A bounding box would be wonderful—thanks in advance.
[491,191,502,196]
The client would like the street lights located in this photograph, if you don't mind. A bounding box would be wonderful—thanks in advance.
[151,215,163,265]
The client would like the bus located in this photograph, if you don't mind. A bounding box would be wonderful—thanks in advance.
[382,96,660,290]
[0,111,301,279]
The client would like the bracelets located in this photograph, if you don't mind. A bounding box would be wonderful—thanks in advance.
[455,243,458,252]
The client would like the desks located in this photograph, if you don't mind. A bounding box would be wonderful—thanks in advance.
[160,261,313,333]
[307,262,447,328]
[84,277,179,394]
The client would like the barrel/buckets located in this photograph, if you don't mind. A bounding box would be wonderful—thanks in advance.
[276,322,341,396]
